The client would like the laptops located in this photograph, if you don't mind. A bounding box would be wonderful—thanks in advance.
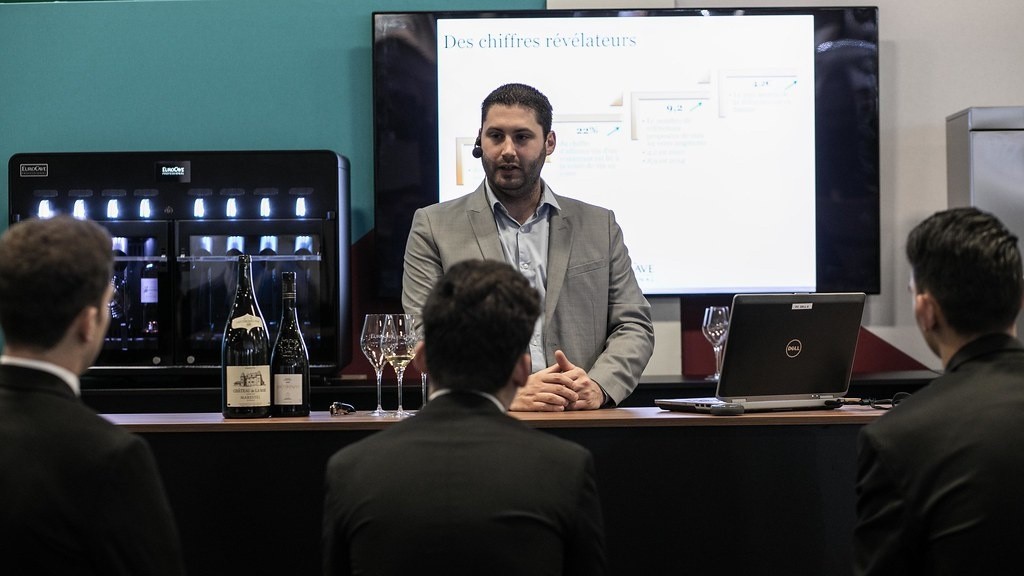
[654,291,866,414]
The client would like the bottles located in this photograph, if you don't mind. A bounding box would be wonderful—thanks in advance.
[139,261,161,331]
[221,255,271,420]
[270,271,310,418]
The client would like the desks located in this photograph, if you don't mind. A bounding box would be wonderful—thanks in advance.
[96,405,898,576]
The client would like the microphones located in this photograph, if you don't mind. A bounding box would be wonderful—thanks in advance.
[473,142,483,158]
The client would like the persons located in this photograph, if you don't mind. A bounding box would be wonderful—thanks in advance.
[852,207,1024,576]
[320,257,607,576]
[0,216,185,576]
[401,84,656,411]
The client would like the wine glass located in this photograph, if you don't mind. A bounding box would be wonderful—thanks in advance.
[406,314,430,411]
[360,314,396,418]
[380,314,419,419]
[702,306,730,379]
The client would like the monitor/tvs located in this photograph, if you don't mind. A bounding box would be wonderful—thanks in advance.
[373,6,882,298]
